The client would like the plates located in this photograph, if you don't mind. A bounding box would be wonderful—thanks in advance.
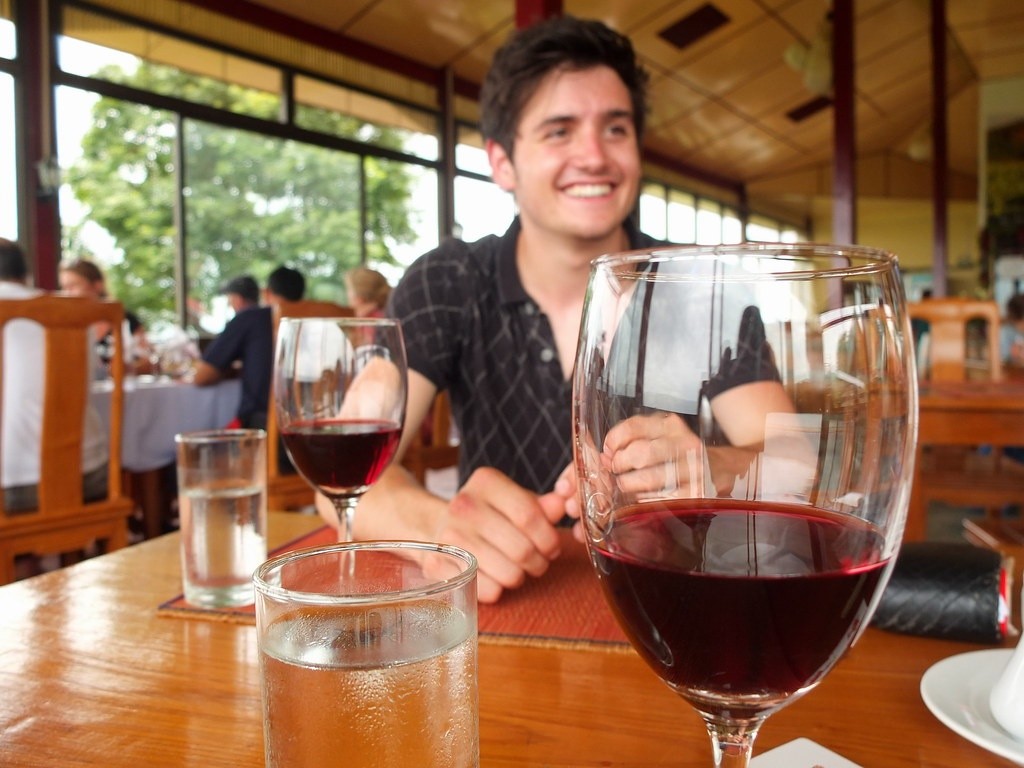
[919,647,1024,767]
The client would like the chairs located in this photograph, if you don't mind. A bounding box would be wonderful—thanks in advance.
[0,291,135,586]
[854,296,1024,544]
[197,293,361,514]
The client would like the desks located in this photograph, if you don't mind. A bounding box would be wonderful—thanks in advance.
[84,374,243,541]
[900,379,1024,541]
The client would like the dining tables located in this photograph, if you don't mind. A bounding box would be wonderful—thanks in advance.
[0,511,1024,768]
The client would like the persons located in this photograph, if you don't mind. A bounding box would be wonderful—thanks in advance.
[0,238,109,581]
[57,261,391,535]
[314,15,818,605]
[912,289,1024,517]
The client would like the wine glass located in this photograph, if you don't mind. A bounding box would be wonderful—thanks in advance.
[275,315,408,589]
[571,243,918,768]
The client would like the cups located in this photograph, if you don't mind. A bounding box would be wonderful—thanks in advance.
[988,630,1024,738]
[251,541,479,768]
[174,428,268,609]
